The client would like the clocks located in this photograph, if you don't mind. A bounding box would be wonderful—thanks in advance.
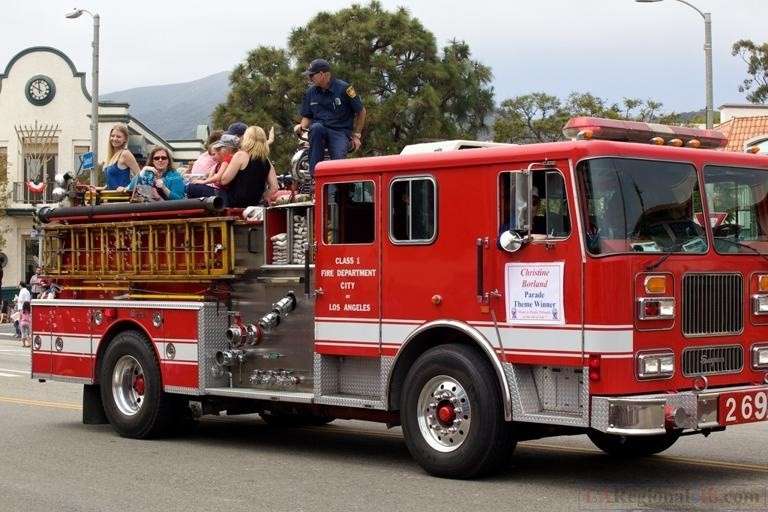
[23,74,56,107]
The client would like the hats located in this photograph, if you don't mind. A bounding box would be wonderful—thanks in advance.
[532,186,540,198]
[212,121,248,151]
[300,59,331,76]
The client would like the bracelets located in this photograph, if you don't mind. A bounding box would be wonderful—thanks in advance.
[354,133,362,138]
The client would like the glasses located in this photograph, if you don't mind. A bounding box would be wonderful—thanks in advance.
[153,156,170,162]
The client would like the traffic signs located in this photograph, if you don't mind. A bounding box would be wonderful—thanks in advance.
[80,151,95,171]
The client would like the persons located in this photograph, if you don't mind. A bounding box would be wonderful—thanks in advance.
[99,123,279,207]
[0,267,54,347]
[497,185,547,251]
[293,58,366,179]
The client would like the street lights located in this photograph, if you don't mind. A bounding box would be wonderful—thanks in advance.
[634,0,715,127]
[62,6,103,195]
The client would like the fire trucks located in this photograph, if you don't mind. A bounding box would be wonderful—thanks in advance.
[21,114,767,480]
